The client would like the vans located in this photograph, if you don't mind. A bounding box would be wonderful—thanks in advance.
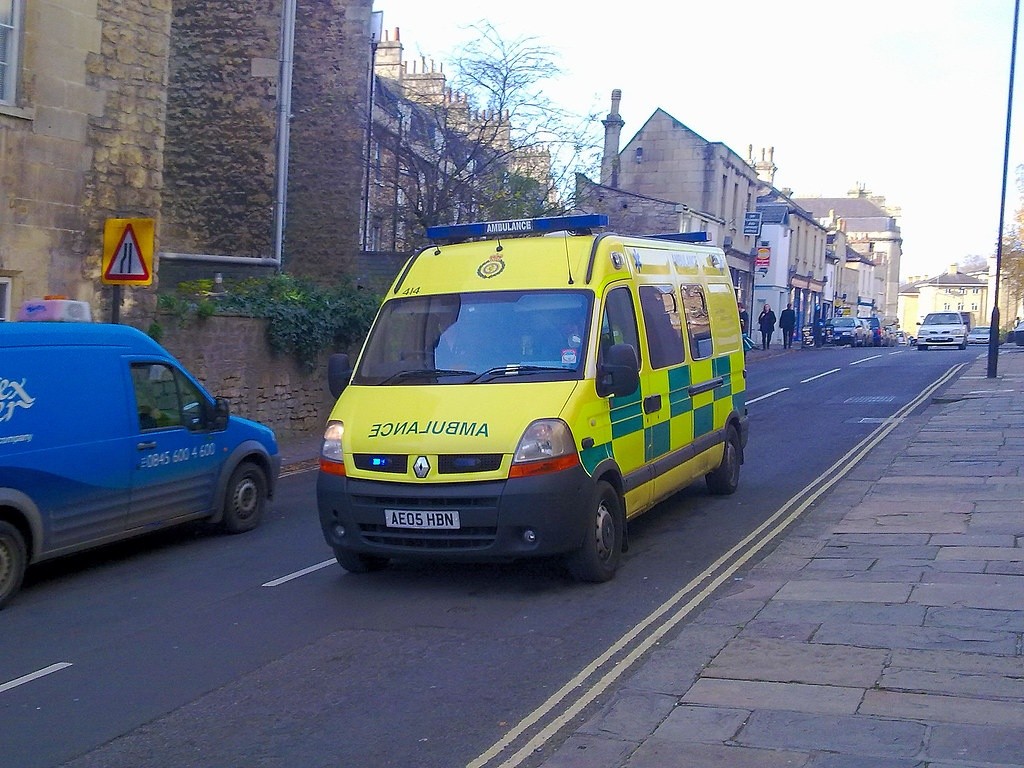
[0,297,280,611]
[313,212,750,584]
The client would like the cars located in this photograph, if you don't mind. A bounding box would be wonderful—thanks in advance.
[916,311,968,351]
[884,324,914,346]
[860,320,874,347]
[967,326,991,343]
[825,318,864,347]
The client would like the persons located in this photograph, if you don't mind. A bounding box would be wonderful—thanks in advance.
[779,303,796,349]
[758,304,776,351]
[738,302,749,338]
[429,311,466,372]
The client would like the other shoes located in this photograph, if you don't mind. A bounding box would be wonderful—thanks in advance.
[784,345,795,349]
[767,344,770,350]
[761,348,766,351]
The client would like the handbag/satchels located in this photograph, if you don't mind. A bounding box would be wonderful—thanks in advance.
[743,334,755,351]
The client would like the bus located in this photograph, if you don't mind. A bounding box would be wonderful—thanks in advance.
[960,311,977,333]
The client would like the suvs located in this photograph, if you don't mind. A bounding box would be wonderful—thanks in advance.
[857,316,885,346]
